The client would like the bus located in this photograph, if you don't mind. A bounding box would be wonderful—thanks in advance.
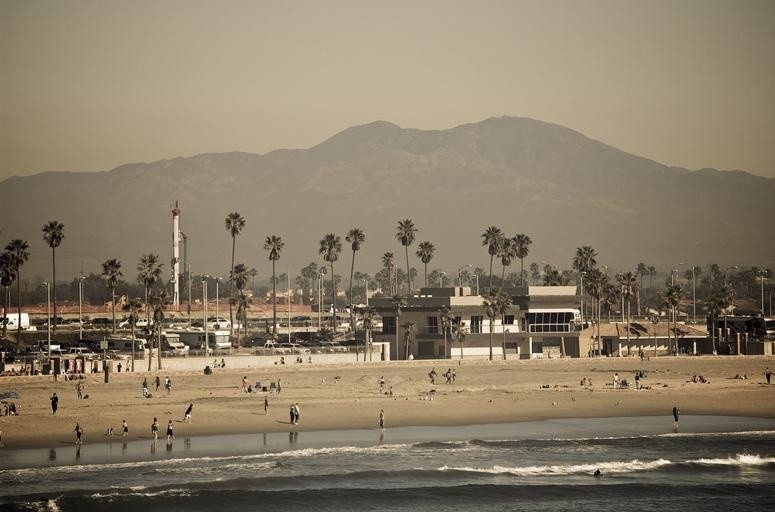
[242,317,277,328]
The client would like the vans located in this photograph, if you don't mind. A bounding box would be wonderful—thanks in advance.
[119,318,153,329]
[316,316,341,327]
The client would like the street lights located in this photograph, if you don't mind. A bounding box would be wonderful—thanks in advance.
[440,272,447,288]
[592,321,597,357]
[724,265,739,315]
[580,272,587,330]
[187,265,191,326]
[672,263,684,354]
[458,264,474,286]
[202,274,210,351]
[691,264,697,324]
[363,279,368,305]
[596,265,609,273]
[318,273,324,330]
[252,265,256,289]
[78,276,87,340]
[213,276,223,323]
[757,270,768,316]
[42,281,50,359]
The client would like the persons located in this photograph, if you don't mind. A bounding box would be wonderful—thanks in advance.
[2,349,193,442]
[430,367,457,385]
[641,352,644,361]
[47,430,300,460]
[212,358,225,368]
[581,372,640,390]
[692,374,707,383]
[766,367,773,383]
[380,409,384,428]
[673,407,679,422]
[241,375,302,425]
[380,376,394,395]
[277,356,313,364]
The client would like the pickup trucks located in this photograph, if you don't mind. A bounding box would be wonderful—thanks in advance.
[82,318,119,329]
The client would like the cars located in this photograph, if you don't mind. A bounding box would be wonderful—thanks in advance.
[247,331,364,356]
[159,318,173,328]
[64,318,85,323]
[206,319,231,328]
[32,319,47,330]
[191,318,203,327]
[718,344,735,355]
[45,317,64,324]
[350,317,378,327]
[174,319,189,326]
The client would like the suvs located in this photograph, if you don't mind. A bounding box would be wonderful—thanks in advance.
[279,315,312,327]
[67,347,99,360]
[27,339,60,352]
[50,349,78,360]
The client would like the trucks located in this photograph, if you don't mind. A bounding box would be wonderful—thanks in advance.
[0,313,29,330]
[704,314,775,341]
[102,350,132,360]
[137,332,185,352]
[100,335,160,360]
